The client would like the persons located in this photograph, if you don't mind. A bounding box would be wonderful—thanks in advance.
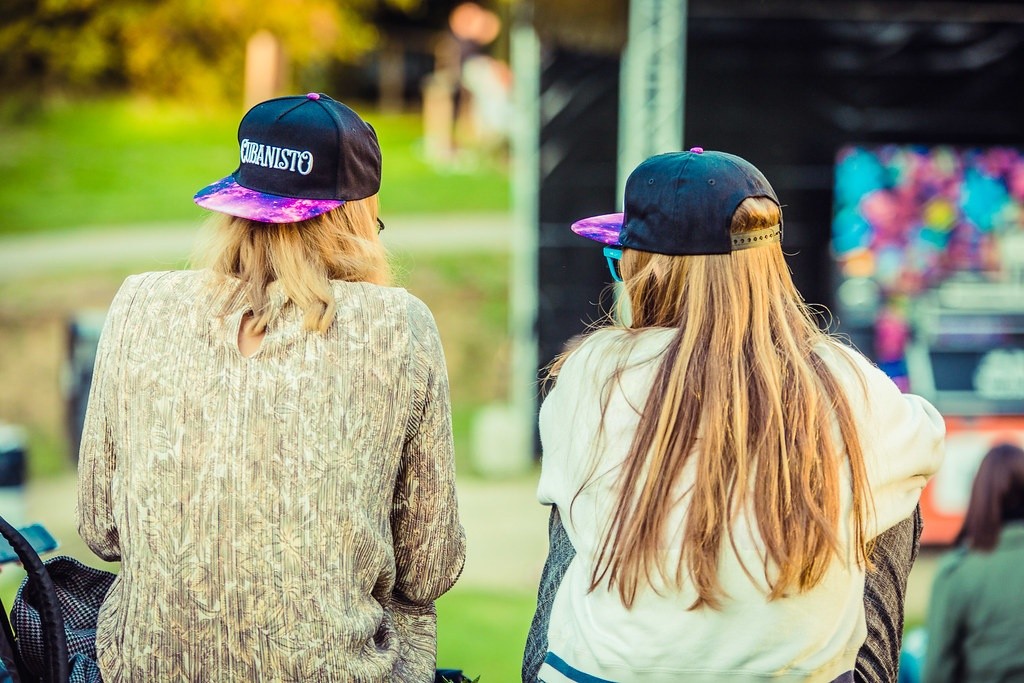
[922,442,1024,683]
[522,148,946,683]
[75,91,466,683]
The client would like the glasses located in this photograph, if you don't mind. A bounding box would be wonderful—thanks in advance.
[377,217,385,234]
[603,244,624,284]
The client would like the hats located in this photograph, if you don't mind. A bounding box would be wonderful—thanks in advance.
[571,147,784,255]
[193,93,382,223]
